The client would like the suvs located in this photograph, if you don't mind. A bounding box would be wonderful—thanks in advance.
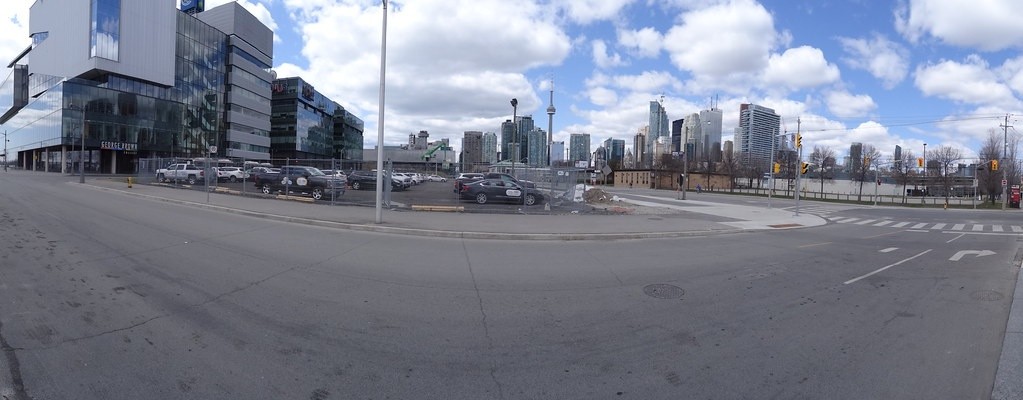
[348,168,428,192]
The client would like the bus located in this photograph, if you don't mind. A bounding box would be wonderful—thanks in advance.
[186,157,234,168]
[236,161,273,170]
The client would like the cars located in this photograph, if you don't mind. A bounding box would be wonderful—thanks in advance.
[453,172,544,206]
[197,166,282,183]
[429,175,446,182]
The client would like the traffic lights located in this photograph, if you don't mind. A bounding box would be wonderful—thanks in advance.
[797,134,802,148]
[992,160,997,170]
[775,164,781,173]
[919,158,923,167]
[802,162,809,174]
[865,156,868,165]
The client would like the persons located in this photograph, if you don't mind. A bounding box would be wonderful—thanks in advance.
[696,183,701,193]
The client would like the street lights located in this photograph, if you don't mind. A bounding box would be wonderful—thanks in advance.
[643,152,657,190]
[682,120,711,199]
[510,98,518,177]
[0,129,9,172]
[921,143,927,205]
[68,103,86,183]
[566,143,583,170]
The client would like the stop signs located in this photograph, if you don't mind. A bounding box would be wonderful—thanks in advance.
[1002,180,1007,185]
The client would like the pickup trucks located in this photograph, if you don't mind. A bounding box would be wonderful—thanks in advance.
[253,165,346,200]
[155,163,204,185]
